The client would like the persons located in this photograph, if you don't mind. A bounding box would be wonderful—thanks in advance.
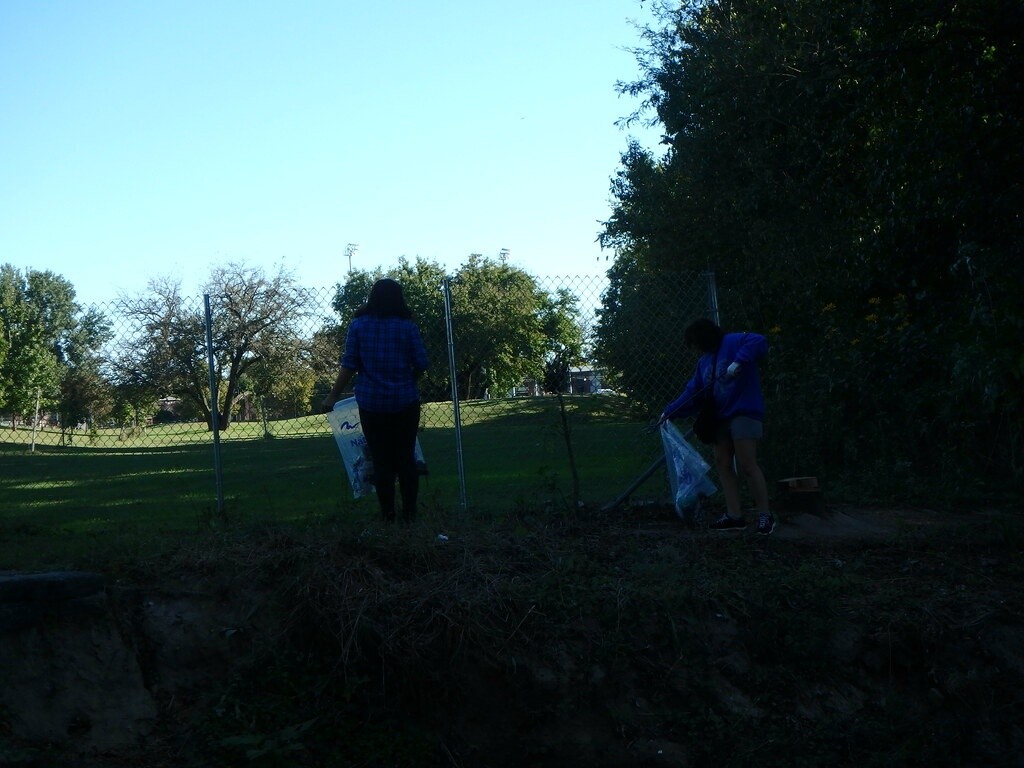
[659,317,776,536]
[320,279,434,528]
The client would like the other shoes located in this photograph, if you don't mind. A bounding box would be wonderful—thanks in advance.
[399,513,416,529]
[376,512,395,524]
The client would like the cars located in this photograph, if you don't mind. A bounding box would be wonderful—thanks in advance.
[516,387,531,396]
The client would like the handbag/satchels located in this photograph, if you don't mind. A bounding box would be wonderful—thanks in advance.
[693,395,718,445]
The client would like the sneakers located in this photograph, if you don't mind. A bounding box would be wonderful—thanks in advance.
[707,513,748,531]
[756,512,776,535]
